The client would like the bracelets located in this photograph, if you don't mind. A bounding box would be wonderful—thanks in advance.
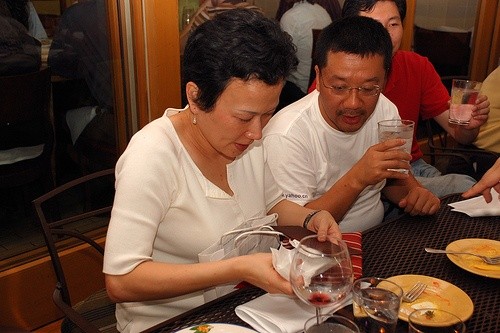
[303,208,321,228]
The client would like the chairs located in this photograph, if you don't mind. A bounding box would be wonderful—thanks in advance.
[0,45,65,239]
[410,22,473,90]
[33,168,120,333]
[424,116,498,178]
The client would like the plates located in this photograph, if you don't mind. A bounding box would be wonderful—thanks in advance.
[376,274,474,327]
[446,239,500,278]
[173,323,259,333]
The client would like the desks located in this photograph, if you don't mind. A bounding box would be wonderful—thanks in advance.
[137,176,500,333]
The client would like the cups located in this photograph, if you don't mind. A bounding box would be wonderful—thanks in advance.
[351,277,404,333]
[376,119,415,176]
[448,79,483,126]
[184,5,194,25]
[304,314,360,333]
[408,308,466,333]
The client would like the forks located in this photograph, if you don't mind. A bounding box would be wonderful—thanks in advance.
[402,281,428,303]
[424,247,500,265]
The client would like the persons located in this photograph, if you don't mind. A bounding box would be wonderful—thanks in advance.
[1,0,115,227]
[102,0,500,333]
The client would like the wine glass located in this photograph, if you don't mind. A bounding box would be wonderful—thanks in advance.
[290,235,354,325]
